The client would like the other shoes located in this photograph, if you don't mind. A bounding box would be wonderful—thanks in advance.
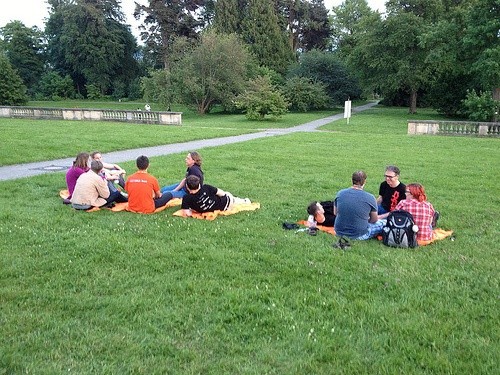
[333,238,351,250]
[309,226,317,235]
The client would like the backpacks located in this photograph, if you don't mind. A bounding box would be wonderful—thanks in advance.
[383,210,417,248]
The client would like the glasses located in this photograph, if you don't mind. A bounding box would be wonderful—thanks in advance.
[405,190,411,193]
[384,175,397,178]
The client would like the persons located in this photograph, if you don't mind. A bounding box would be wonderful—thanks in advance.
[124,155,173,213]
[158,152,204,198]
[307,166,439,241]
[66,151,126,200]
[69,160,128,211]
[181,176,251,216]
